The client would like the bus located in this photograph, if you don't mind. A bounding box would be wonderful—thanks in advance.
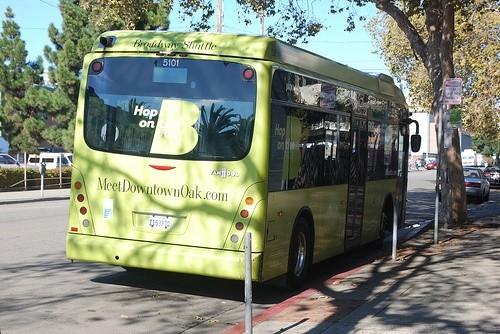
[67,30,421,288]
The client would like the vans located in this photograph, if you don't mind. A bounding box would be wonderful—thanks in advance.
[27,152,64,169]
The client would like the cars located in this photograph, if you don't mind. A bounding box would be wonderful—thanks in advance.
[0,155,33,170]
[463,168,491,202]
